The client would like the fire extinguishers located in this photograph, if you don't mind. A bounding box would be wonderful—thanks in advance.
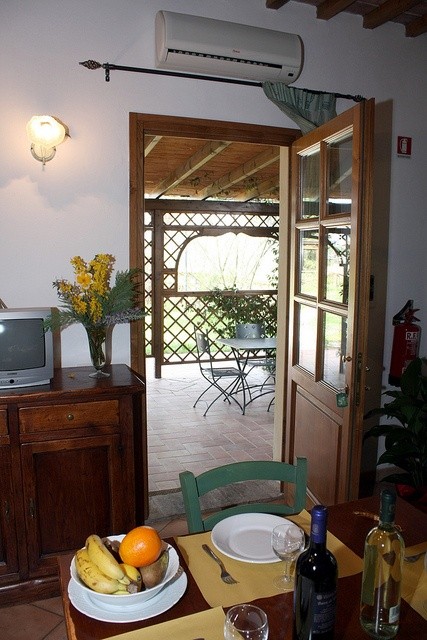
[389,299,422,387]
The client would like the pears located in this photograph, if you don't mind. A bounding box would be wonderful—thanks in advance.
[139,547,172,588]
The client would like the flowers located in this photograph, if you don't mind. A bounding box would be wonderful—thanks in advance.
[37,251,150,337]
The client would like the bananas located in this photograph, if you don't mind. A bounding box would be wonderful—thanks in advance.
[75,534,139,595]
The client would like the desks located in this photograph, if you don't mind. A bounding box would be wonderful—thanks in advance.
[213,338,276,416]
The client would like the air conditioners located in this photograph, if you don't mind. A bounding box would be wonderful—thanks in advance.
[154,9,302,87]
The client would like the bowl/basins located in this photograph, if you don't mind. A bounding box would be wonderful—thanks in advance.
[69,533,179,607]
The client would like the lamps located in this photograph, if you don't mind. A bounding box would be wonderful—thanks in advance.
[26,115,67,168]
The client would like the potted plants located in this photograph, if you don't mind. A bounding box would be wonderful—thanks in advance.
[361,356,426,513]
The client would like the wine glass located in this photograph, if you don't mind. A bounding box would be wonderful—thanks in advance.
[271,523,305,591]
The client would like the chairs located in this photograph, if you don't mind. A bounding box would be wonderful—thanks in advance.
[191,326,247,418]
[234,322,276,394]
[177,455,307,534]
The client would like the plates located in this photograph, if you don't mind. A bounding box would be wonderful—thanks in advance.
[209,512,308,565]
[66,565,188,624]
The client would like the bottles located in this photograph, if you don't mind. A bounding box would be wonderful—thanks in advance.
[356,486,405,640]
[291,503,338,640]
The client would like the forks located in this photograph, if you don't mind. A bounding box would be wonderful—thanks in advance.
[200,543,238,585]
[401,551,426,563]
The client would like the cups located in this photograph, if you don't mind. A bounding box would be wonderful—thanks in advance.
[223,604,269,639]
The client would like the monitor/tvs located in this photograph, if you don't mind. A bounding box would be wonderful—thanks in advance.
[1,306,55,389]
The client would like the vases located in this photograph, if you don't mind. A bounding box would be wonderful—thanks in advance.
[80,322,111,379]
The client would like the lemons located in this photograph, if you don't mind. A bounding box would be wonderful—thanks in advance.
[113,564,142,594]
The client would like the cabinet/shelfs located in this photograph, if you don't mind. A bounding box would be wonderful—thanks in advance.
[0,363,146,609]
